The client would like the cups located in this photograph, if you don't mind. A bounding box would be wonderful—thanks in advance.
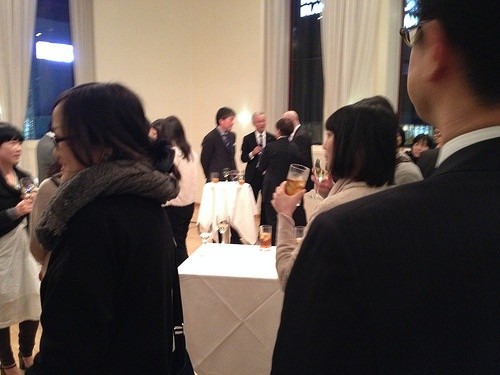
[294,226,304,246]
[237,170,245,185]
[259,225,272,251]
[285,164,310,207]
[211,172,219,182]
[20,177,39,200]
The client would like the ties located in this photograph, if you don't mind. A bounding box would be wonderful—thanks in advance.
[256,135,263,168]
[222,134,231,150]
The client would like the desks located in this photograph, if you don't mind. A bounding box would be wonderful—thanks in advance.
[196,182,260,244]
[177,243,284,375]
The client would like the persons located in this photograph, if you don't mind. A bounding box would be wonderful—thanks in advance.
[271,95,425,293]
[240,110,277,203]
[397,125,443,179]
[201,106,243,245]
[26,81,187,375]
[150,116,198,266]
[270,0,500,375]
[30,157,63,279]
[258,118,303,246]
[0,120,39,375]
[36,121,61,186]
[281,111,314,227]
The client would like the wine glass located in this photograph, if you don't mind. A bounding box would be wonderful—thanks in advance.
[216,215,231,245]
[223,168,231,182]
[197,220,214,247]
[230,170,239,182]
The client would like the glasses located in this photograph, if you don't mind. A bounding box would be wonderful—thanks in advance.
[50,134,71,154]
[398,16,436,47]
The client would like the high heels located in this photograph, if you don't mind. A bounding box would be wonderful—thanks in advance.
[0,361,17,375]
[18,351,33,370]
